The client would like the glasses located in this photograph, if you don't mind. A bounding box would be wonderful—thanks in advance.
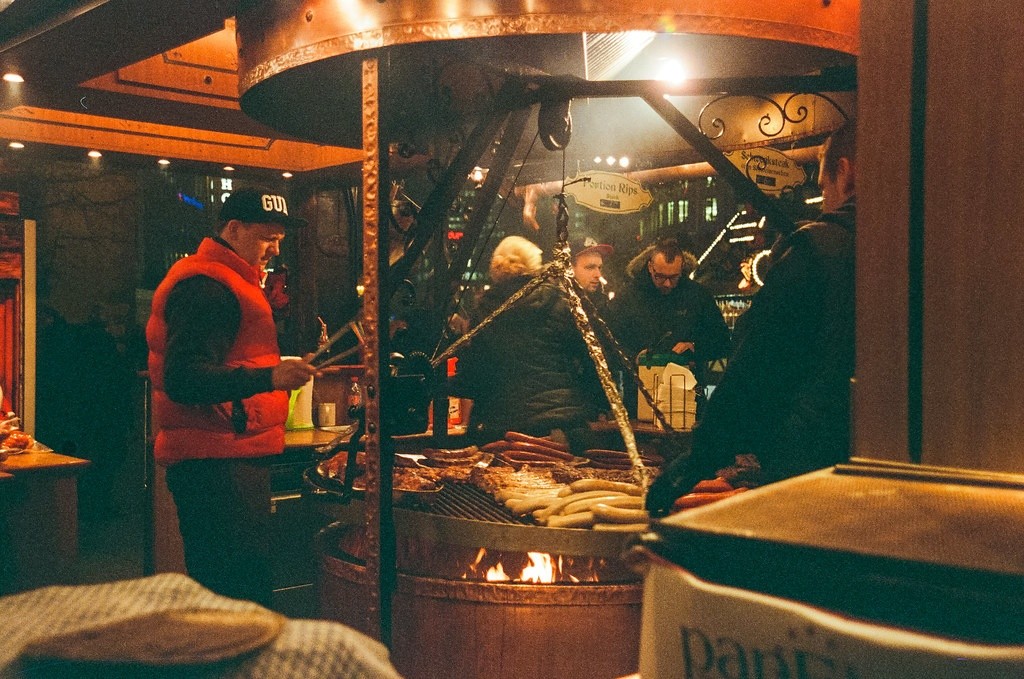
[650,265,681,282]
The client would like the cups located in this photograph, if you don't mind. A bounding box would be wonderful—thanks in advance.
[317,403,336,426]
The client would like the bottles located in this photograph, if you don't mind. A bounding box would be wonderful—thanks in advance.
[712,298,754,335]
[347,377,362,428]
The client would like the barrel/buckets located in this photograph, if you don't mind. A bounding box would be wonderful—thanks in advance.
[637,335,691,422]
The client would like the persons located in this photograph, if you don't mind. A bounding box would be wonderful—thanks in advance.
[644,124,854,517]
[470,235,588,441]
[567,233,623,421]
[603,238,734,422]
[144,184,322,614]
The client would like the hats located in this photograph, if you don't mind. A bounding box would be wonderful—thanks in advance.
[220,186,309,230]
[570,237,613,257]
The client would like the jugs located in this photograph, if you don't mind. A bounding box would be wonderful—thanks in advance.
[288,374,314,429]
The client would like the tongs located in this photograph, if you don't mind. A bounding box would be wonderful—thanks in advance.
[302,313,373,371]
